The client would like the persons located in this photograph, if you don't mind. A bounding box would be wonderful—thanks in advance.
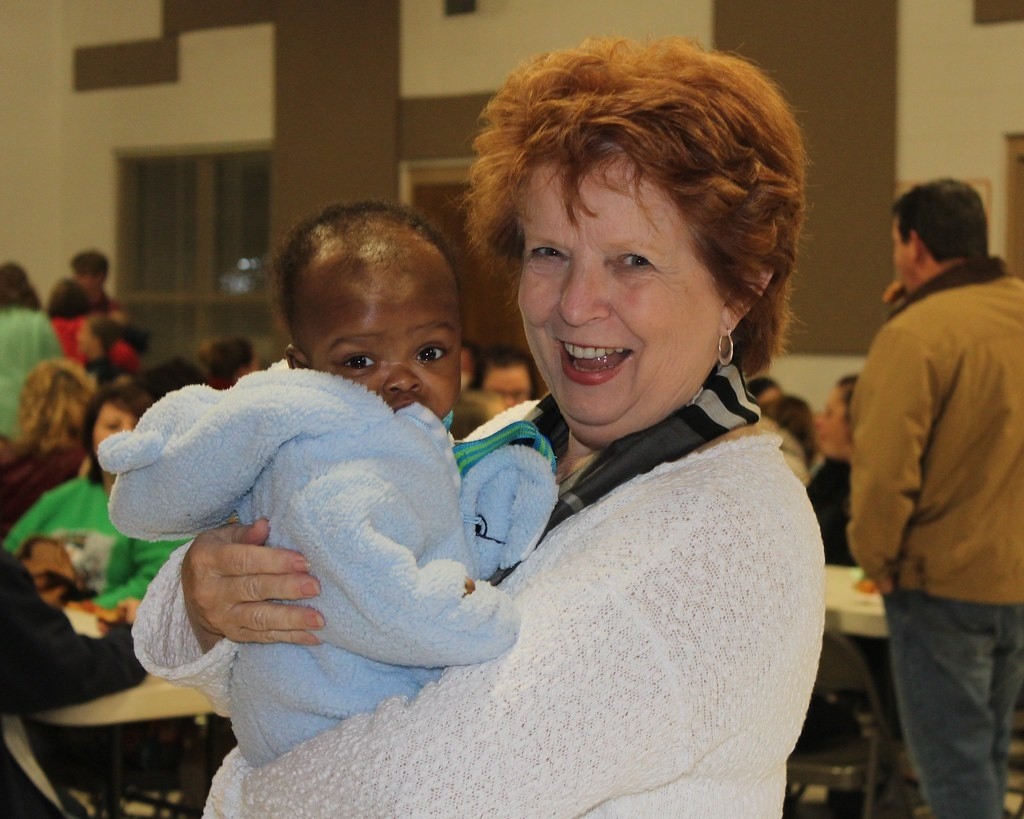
[744,374,915,780]
[96,201,556,768]
[843,176,1024,819]
[200,34,824,817]
[0,240,537,819]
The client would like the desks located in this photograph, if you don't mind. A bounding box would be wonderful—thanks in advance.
[779,561,906,819]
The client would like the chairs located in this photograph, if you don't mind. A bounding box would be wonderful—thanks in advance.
[15,455,220,819]
